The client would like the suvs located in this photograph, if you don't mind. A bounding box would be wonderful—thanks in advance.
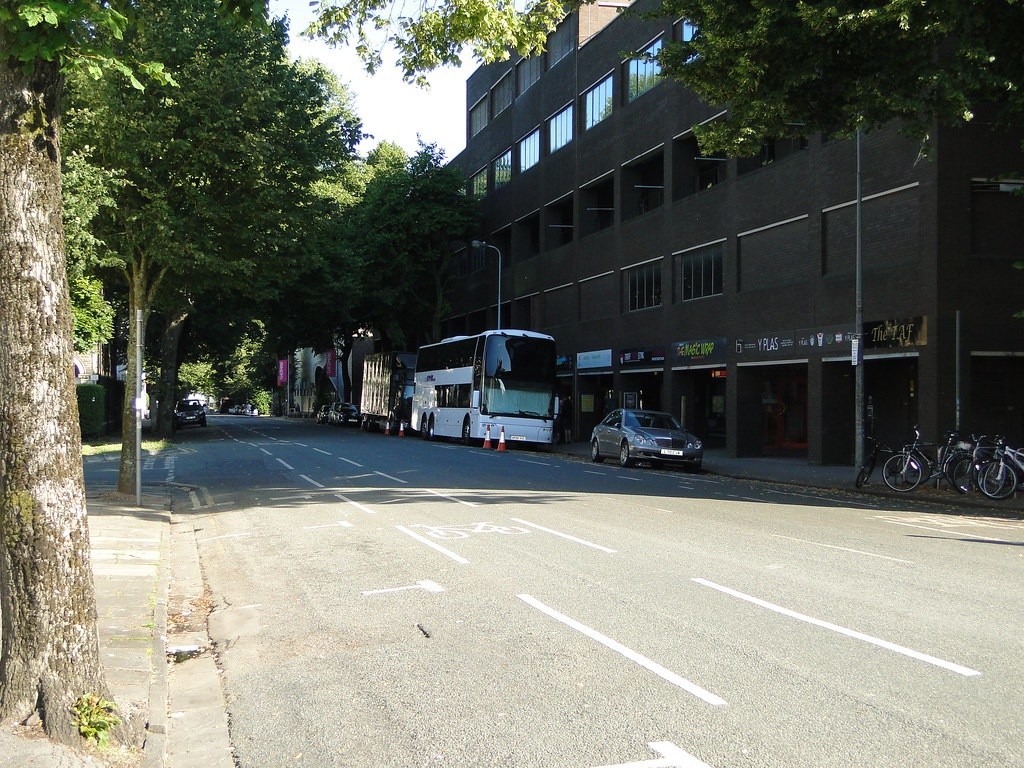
[328,401,361,427]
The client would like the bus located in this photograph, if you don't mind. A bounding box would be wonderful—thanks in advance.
[410,329,559,447]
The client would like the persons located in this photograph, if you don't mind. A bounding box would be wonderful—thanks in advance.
[557,393,572,442]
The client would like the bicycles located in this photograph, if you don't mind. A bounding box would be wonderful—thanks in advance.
[856,436,934,490]
[882,423,1024,501]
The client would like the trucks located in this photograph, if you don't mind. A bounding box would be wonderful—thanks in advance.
[360,350,416,436]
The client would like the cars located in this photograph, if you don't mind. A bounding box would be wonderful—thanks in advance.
[207,402,259,417]
[173,399,207,430]
[590,407,704,474]
[315,404,331,424]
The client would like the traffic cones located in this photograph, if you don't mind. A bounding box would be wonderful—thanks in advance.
[359,420,366,432]
[397,422,406,438]
[493,425,510,453]
[384,421,391,436]
[481,423,492,450]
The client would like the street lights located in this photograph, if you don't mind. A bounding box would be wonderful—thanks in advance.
[472,240,502,331]
